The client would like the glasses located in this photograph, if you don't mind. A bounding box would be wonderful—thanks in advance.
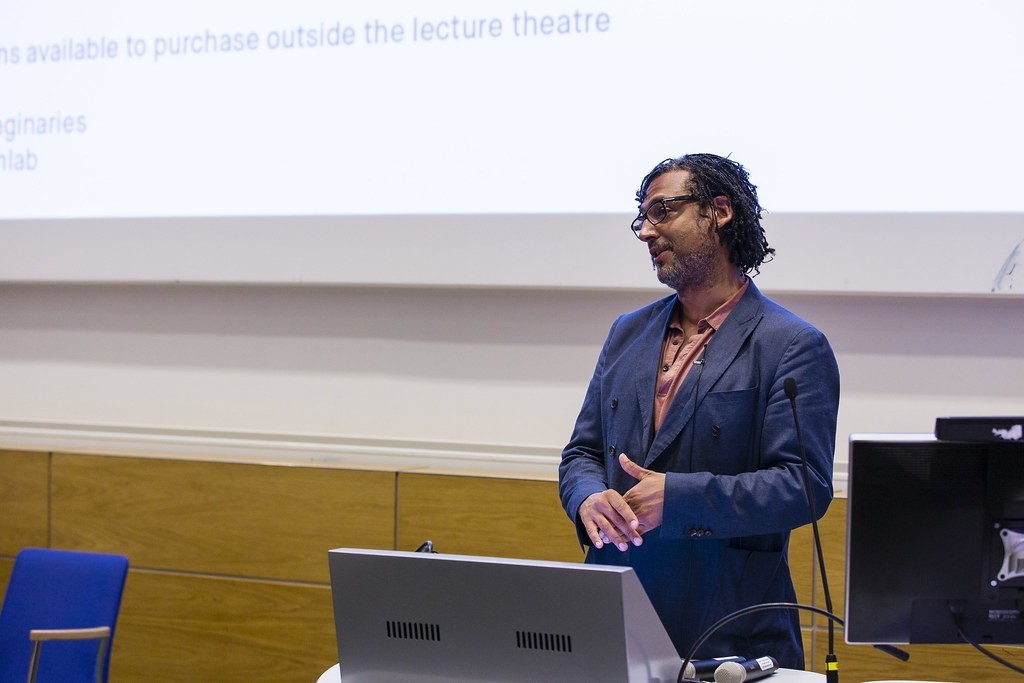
[631,194,716,239]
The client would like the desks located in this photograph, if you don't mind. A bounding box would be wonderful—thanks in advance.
[316,661,827,683]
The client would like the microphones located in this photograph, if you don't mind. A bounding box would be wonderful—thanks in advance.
[783,377,839,683]
[681,656,747,681]
[713,656,780,683]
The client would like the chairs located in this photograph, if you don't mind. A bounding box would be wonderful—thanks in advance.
[0,549,128,683]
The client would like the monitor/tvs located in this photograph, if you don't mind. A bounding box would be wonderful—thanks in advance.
[846,432,1023,648]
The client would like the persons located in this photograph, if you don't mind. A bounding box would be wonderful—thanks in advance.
[558,153,841,671]
